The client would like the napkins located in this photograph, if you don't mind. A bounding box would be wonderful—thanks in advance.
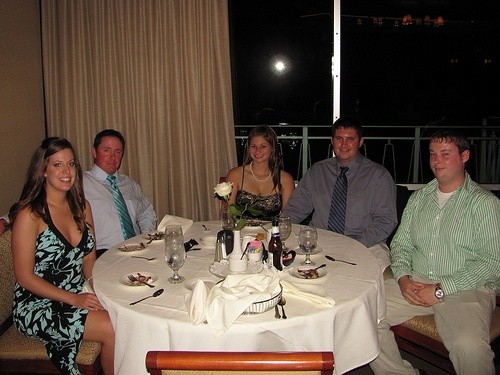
[185,274,281,339]
[157,214,194,235]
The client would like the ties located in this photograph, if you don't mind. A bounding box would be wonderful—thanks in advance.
[328,167,349,235]
[106,175,136,241]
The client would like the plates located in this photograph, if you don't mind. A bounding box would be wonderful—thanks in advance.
[208,259,264,278]
[243,220,272,229]
[120,272,158,287]
[120,243,148,253]
[141,231,164,242]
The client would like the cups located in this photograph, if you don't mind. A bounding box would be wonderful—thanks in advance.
[164,224,184,248]
[229,254,247,271]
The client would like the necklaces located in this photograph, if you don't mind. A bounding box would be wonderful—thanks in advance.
[250,162,271,182]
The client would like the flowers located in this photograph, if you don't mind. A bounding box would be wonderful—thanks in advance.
[213,180,268,231]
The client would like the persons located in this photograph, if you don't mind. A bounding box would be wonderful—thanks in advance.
[369,127,500,375]
[3,137,115,375]
[281,116,398,247]
[0,128,158,260]
[225,126,295,221]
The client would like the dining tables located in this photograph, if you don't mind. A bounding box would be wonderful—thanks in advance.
[93,220,387,375]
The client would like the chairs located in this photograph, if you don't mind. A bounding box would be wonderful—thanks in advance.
[145,350,336,375]
[0,177,500,375]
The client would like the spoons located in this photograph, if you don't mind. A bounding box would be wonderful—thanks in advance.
[130,288,164,306]
[325,255,357,266]
[278,297,287,320]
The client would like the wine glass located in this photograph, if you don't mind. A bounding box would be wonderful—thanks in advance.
[273,214,318,266]
[165,242,185,284]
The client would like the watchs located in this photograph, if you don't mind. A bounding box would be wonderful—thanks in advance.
[435,284,443,299]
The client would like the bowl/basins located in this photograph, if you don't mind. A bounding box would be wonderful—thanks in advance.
[216,279,283,314]
[287,266,329,286]
[200,235,217,250]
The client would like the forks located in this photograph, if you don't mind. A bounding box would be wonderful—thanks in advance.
[127,275,155,289]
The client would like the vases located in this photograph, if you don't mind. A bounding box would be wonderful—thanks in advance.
[227,230,248,261]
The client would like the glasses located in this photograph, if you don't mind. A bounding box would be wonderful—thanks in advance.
[333,136,360,143]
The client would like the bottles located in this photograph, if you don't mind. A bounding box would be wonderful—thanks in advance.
[215,227,234,260]
[268,219,282,272]
[240,233,266,259]
